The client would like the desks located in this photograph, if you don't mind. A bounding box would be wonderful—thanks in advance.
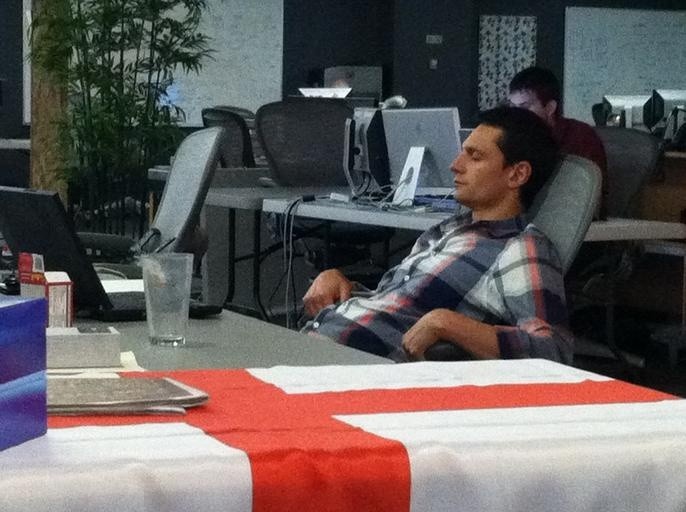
[147,163,171,182]
[0,277,686,512]
[262,189,686,380]
[193,183,352,316]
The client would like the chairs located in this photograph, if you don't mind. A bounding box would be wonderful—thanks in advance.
[454,154,602,277]
[214,105,268,168]
[76,124,227,299]
[254,96,354,189]
[201,108,257,169]
[569,126,663,351]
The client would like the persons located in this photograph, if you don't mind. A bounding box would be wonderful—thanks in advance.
[299,107,575,367]
[509,66,608,174]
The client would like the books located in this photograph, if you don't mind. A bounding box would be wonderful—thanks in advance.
[45,377,209,413]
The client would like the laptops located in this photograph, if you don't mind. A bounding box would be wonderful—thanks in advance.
[1,184,223,322]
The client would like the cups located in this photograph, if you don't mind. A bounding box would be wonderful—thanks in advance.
[142,254,193,348]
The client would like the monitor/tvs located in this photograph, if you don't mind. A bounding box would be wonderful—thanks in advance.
[343,106,389,200]
[652,88,686,143]
[380,106,462,212]
[601,94,651,134]
